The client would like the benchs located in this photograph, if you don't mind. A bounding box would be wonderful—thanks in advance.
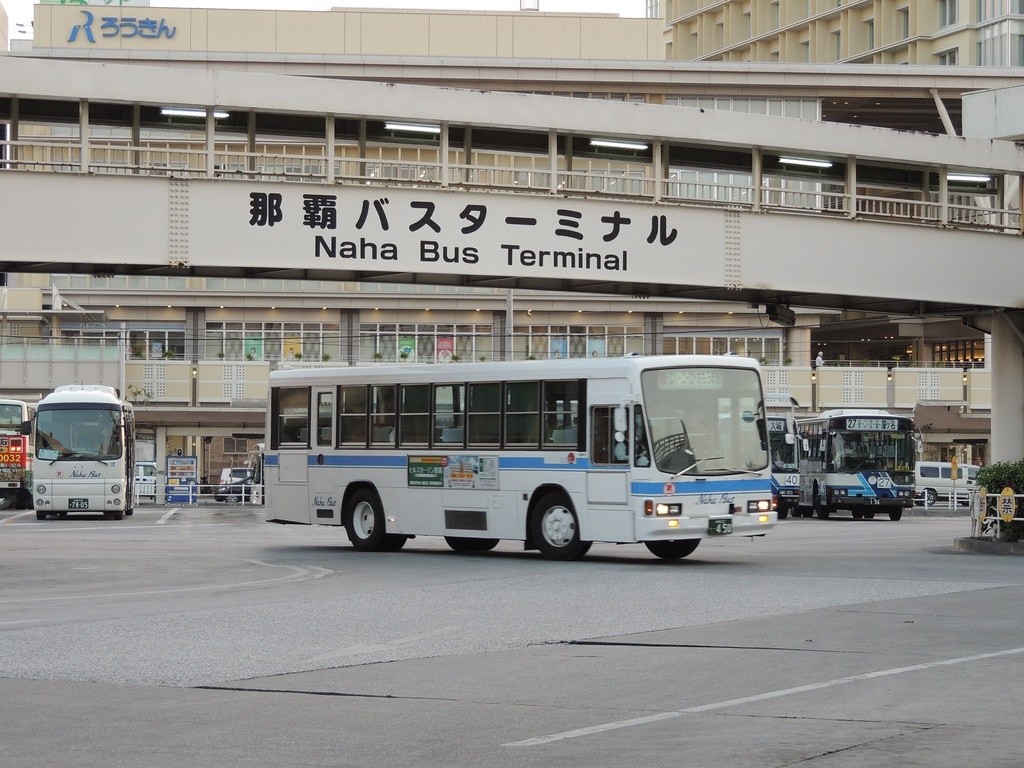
[296,427,308,442]
[319,427,332,444]
[545,425,578,443]
[439,425,464,443]
[371,426,393,442]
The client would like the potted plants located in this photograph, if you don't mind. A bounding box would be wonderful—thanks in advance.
[162,350,176,361]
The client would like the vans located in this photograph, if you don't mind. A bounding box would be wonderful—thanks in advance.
[136,459,157,501]
[914,461,986,507]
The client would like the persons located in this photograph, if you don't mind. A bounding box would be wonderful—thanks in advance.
[815,351,825,368]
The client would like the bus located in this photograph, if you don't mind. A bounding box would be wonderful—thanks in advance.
[767,414,810,520]
[263,353,800,564]
[795,408,926,523]
[0,399,35,508]
[16,382,137,522]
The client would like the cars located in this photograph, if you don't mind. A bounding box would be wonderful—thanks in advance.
[213,475,255,504]
[218,467,255,492]
